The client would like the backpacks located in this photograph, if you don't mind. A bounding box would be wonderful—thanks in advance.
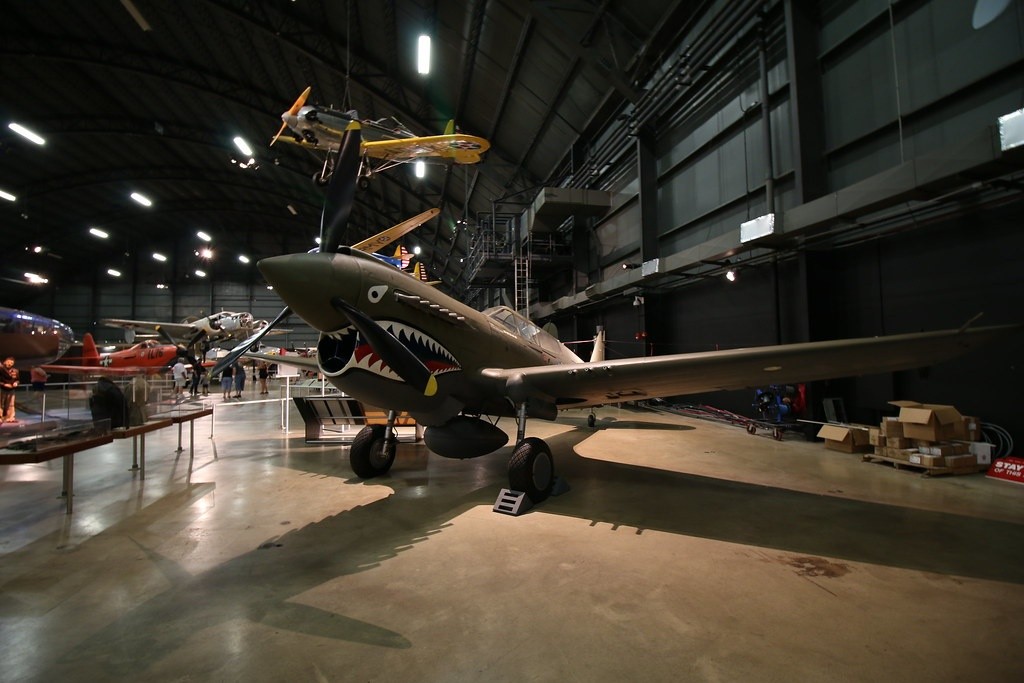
[124,376,150,427]
[88,377,129,431]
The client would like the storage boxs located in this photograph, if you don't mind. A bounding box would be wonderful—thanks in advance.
[816,400,979,476]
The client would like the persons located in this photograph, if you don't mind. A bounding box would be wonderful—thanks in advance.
[189,358,209,394]
[258,360,269,394]
[171,357,189,400]
[199,367,210,395]
[221,366,233,399]
[0,356,20,422]
[32,361,48,399]
[233,360,246,398]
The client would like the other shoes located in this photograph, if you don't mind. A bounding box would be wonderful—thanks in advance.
[180,397,184,399]
[175,395,178,398]
[189,390,192,394]
[238,394,240,397]
[260,392,264,394]
[195,391,198,394]
[223,396,225,398]
[207,391,209,393]
[228,396,230,398]
[203,392,205,393]
[233,396,238,398]
[265,392,268,394]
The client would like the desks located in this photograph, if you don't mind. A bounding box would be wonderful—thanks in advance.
[81,418,173,480]
[146,409,213,454]
[0,431,114,513]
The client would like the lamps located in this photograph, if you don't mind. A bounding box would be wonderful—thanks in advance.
[726,268,736,281]
[287,204,298,215]
[120,0,152,32]
[633,296,645,306]
[622,263,642,269]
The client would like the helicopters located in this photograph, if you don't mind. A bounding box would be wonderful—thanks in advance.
[41,330,220,374]
[0,299,233,424]
[264,84,490,189]
[223,253,1024,518]
[97,309,294,353]
[211,208,442,381]
